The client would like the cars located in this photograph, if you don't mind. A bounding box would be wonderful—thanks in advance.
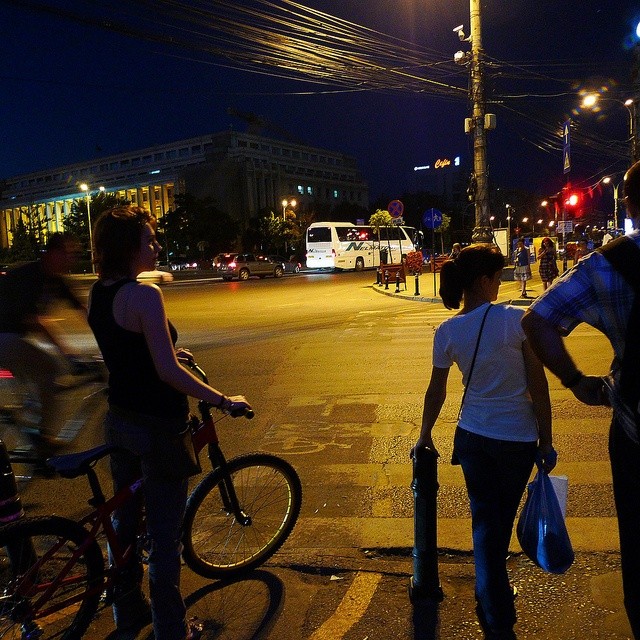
[258,256,300,274]
[167,256,220,271]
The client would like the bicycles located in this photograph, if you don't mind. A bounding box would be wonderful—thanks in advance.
[0,346,304,640]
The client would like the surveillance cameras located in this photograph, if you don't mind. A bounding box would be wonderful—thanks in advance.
[453,24,463,33]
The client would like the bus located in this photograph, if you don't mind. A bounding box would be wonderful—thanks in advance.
[305,221,424,273]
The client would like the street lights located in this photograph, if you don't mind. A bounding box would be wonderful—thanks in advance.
[79,183,95,275]
[281,199,297,254]
[583,96,633,138]
[603,174,628,235]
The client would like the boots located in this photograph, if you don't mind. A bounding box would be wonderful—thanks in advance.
[112,591,153,636]
[154,608,204,640]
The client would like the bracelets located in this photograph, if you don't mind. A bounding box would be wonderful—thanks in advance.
[557,364,583,390]
[214,390,233,415]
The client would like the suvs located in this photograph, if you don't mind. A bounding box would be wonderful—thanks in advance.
[220,253,285,281]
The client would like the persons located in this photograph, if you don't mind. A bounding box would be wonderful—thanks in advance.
[519,162,640,640]
[449,243,460,261]
[573,238,590,266]
[513,236,532,297]
[535,237,558,289]
[85,202,254,640]
[411,241,558,639]
[0,221,87,462]
[598,229,613,247]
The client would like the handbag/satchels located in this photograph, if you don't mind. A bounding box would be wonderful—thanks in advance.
[450,421,461,465]
